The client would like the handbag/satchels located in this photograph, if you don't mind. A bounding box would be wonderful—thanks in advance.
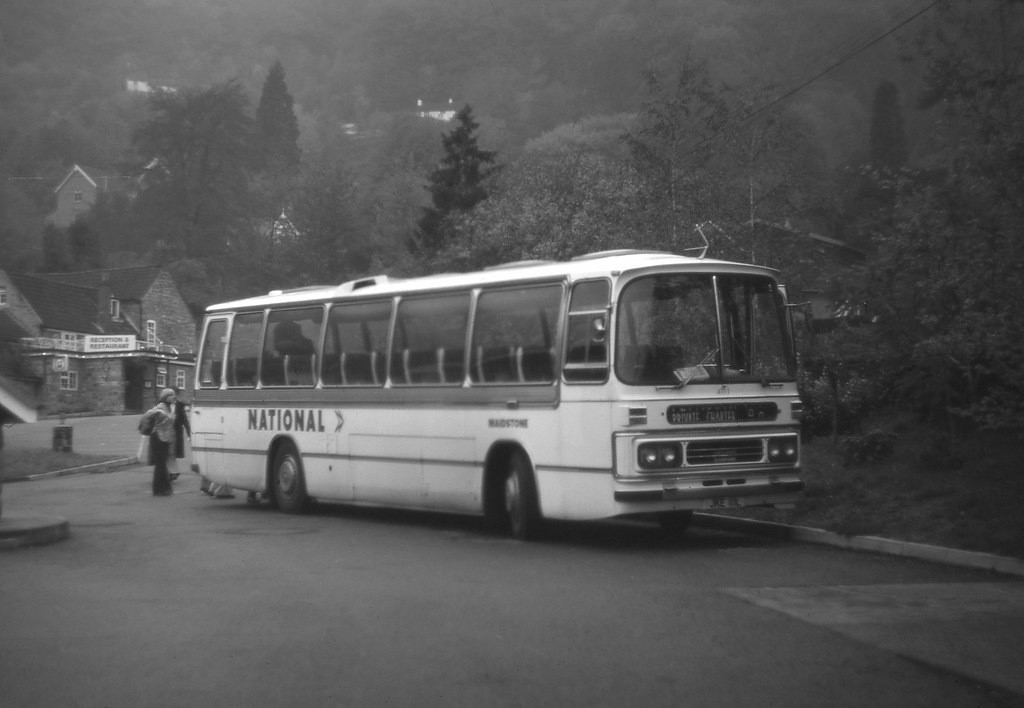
[138,410,157,435]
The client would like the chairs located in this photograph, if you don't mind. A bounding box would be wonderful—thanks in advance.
[210,337,560,382]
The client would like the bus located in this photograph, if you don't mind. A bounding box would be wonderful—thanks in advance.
[189,247,804,541]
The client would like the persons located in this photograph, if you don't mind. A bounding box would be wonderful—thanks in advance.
[139,388,177,496]
[163,386,193,483]
